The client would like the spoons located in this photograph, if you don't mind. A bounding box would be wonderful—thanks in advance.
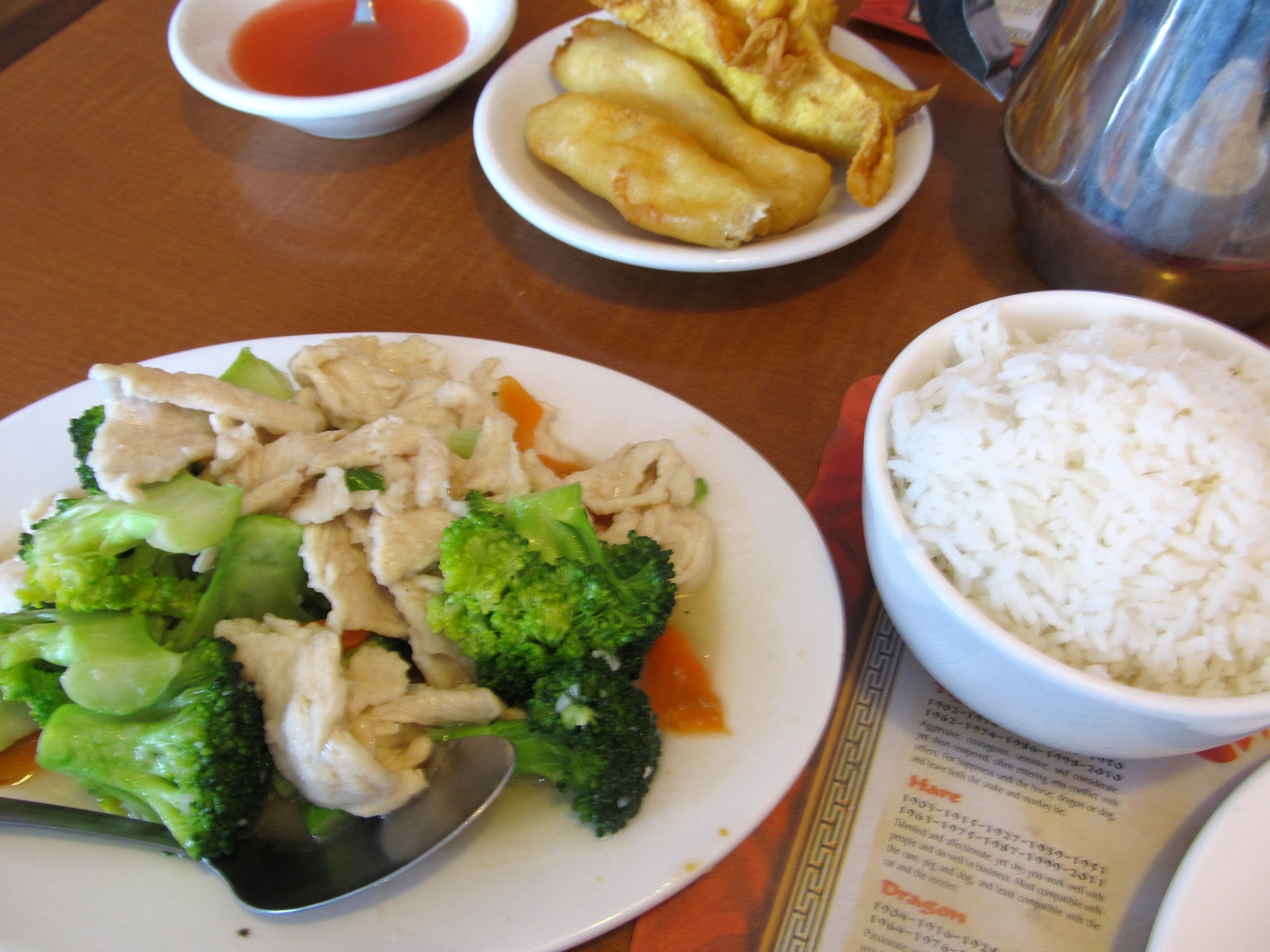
[2,732,514,912]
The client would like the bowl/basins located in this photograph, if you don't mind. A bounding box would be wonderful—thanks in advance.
[1126,124,1266,256]
[862,291,1270,757]
[167,0,519,138]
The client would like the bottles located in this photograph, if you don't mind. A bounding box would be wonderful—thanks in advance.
[1001,0,1270,328]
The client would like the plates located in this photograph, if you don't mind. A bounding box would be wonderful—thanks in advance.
[0,329,844,952]
[1146,760,1270,951]
[1004,104,1074,185]
[472,8,933,272]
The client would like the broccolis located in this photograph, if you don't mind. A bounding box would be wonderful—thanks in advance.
[0,345,687,867]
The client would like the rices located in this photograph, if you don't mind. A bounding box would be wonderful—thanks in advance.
[882,305,1270,696]
